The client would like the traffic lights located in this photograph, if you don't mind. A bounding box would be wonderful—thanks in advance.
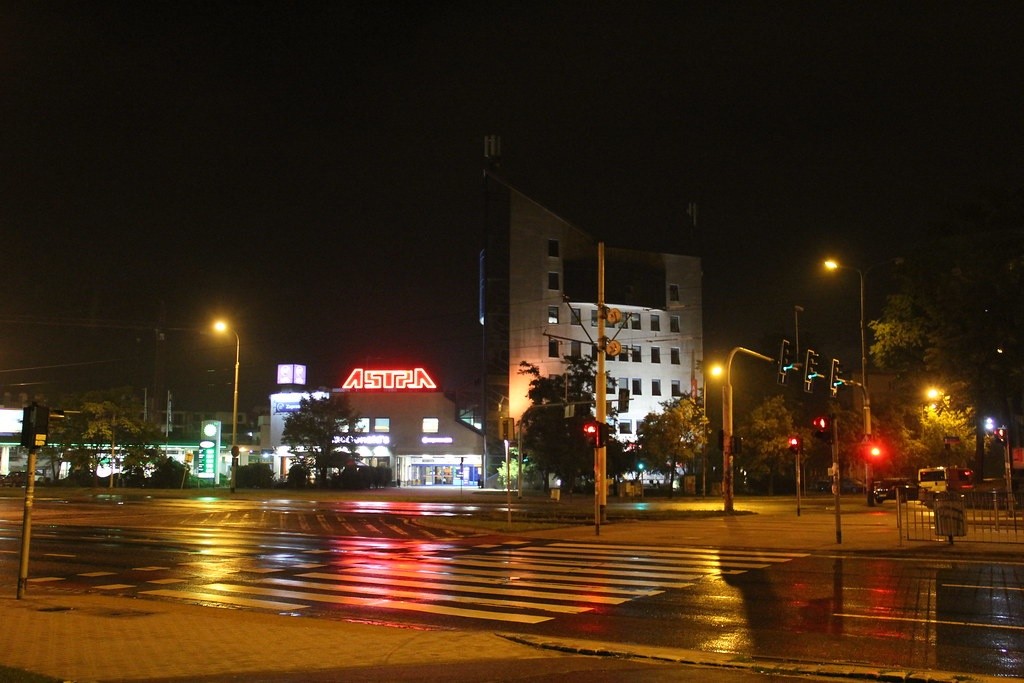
[776,340,793,384]
[996,428,1007,442]
[830,359,842,391]
[583,422,600,448]
[789,436,801,450]
[867,445,882,462]
[638,462,643,470]
[803,349,818,392]
[815,417,830,432]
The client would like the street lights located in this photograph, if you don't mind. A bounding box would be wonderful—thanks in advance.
[215,320,239,493]
[827,257,874,505]
[702,366,722,498]
[920,391,935,444]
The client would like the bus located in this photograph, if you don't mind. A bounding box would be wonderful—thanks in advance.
[918,466,973,500]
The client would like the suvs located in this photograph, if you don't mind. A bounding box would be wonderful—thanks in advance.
[874,478,918,504]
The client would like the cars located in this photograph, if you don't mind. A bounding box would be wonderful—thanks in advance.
[0,470,44,488]
[817,478,865,494]
